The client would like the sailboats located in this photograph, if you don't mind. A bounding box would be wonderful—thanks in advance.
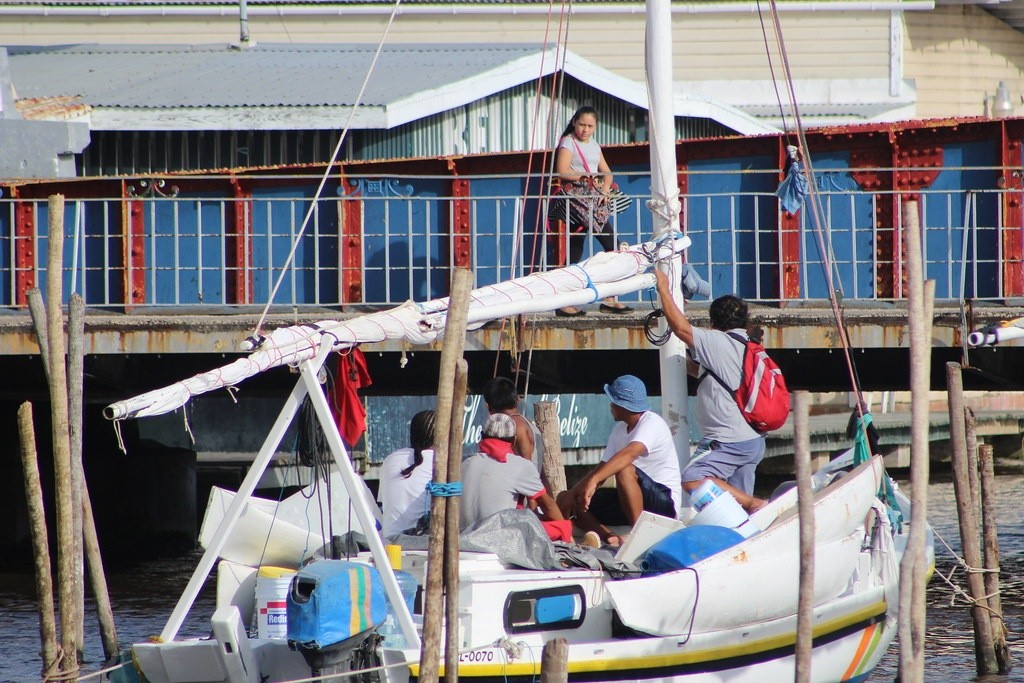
[115,1,936,683]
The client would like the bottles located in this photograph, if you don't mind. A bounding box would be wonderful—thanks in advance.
[377,543,417,643]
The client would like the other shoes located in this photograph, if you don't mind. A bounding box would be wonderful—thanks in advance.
[556,309,585,317]
[600,304,634,313]
[576,531,601,549]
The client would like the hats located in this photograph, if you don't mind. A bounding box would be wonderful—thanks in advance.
[481,413,516,438]
[604,375,652,412]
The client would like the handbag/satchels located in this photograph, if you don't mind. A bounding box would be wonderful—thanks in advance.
[548,176,632,232]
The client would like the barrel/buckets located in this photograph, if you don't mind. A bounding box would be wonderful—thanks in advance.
[257,576,292,642]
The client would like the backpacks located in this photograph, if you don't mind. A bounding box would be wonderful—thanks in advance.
[703,332,791,433]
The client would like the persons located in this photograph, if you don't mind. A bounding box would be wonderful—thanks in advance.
[556,375,681,547]
[459,413,601,549]
[650,268,767,513]
[483,376,542,518]
[556,107,634,317]
[377,409,436,538]
